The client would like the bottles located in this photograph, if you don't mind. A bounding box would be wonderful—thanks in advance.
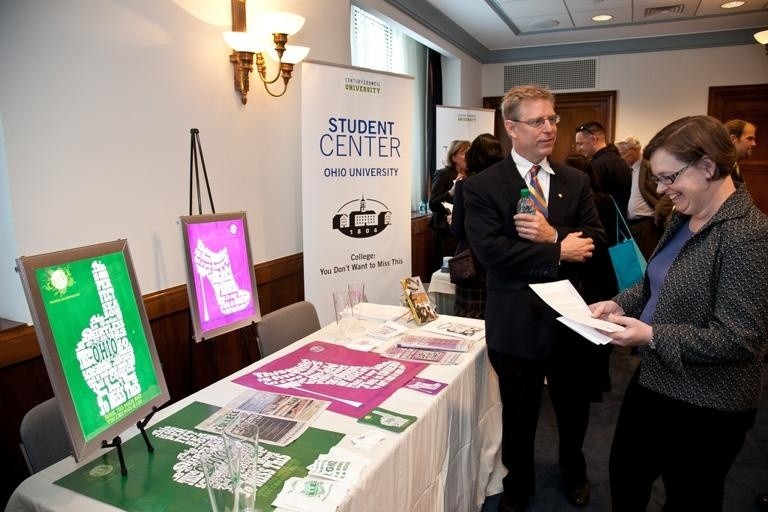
[420,202,425,215]
[516,189,536,216]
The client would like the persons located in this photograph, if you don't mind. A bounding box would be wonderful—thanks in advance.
[462,84,607,512]
[584,114,768,512]
[431,132,504,317]
[569,121,671,401]
[723,116,757,184]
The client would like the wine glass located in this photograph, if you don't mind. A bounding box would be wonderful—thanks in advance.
[332,279,366,344]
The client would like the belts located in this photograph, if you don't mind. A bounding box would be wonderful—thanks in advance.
[629,217,650,224]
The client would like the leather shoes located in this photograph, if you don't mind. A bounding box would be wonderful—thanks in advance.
[564,478,590,507]
[498,492,529,512]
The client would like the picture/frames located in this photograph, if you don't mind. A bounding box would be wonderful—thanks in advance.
[179,211,261,344]
[15,238,171,462]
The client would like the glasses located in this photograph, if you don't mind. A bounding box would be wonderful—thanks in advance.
[513,114,561,128]
[651,159,693,185]
[580,124,593,135]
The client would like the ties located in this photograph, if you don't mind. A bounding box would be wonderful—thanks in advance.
[529,166,548,221]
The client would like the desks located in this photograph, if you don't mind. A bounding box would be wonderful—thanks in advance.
[428,269,457,316]
[4,301,510,512]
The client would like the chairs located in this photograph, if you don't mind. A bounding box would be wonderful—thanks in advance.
[257,301,321,359]
[20,397,73,475]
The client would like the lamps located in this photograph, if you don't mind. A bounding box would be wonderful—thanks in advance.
[754,29,768,55]
[224,0,309,106]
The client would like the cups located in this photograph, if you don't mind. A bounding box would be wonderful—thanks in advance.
[195,420,258,512]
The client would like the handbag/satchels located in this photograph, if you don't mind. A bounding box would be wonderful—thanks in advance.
[448,248,477,284]
[609,236,648,293]
